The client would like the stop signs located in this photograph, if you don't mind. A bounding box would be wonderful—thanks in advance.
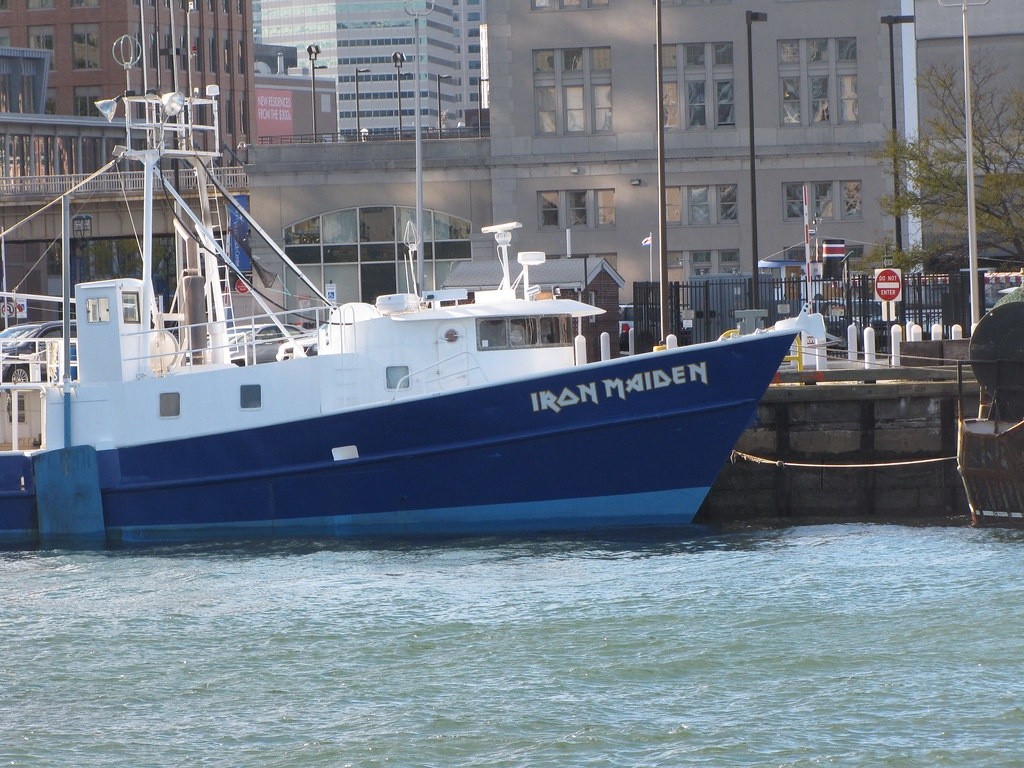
[234,278,250,295]
[875,267,902,302]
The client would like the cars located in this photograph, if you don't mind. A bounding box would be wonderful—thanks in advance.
[0,318,76,385]
[617,302,691,346]
[224,324,308,364]
[813,299,894,347]
[276,315,334,359]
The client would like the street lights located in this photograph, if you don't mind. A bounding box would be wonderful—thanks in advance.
[73,214,93,280]
[438,75,451,139]
[306,45,328,143]
[746,12,769,306]
[478,79,491,137]
[355,68,371,142]
[881,16,918,254]
[391,51,408,140]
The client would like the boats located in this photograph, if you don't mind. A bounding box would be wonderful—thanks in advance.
[955,280,1023,528]
[0,31,812,549]
